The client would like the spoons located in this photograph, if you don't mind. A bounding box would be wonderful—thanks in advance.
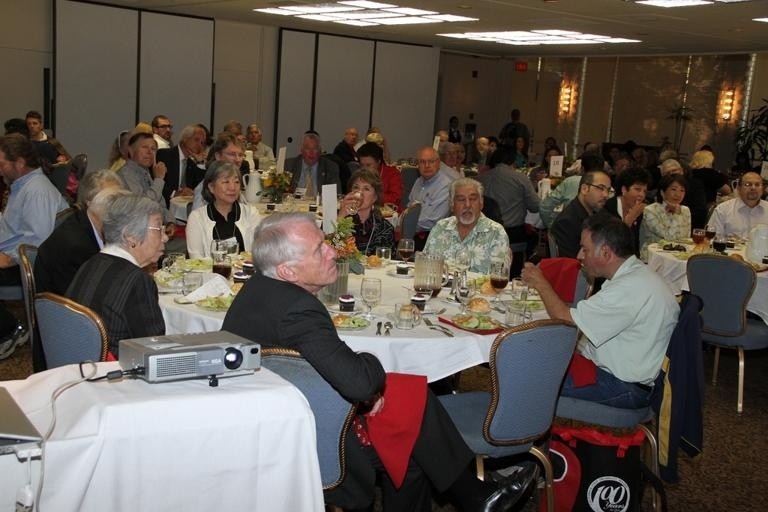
[384,321,392,337]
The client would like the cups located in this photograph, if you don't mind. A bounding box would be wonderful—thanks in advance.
[162,253,184,274]
[376,248,392,265]
[504,306,525,327]
[511,277,528,300]
[176,274,203,296]
[394,301,422,330]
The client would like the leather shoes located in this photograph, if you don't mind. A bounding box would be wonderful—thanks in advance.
[470,462,538,512]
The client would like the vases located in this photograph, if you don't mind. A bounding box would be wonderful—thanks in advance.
[320,261,349,305]
[269,192,283,210]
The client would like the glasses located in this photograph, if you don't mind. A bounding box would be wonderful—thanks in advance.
[220,150,246,160]
[156,124,171,129]
[147,224,168,236]
[743,182,763,187]
[586,182,616,195]
[351,183,374,193]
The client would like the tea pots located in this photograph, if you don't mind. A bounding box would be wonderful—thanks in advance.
[241,171,264,204]
[744,222,768,265]
[536,176,552,201]
[414,252,449,297]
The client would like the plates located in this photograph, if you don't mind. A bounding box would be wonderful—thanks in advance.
[419,305,436,314]
[330,315,370,332]
[327,305,360,314]
[387,271,416,277]
[441,313,508,335]
[193,294,233,313]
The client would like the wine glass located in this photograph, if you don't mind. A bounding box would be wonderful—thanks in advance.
[212,256,232,278]
[282,193,293,211]
[396,239,415,265]
[713,238,726,253]
[361,278,382,321]
[209,239,228,264]
[491,262,511,305]
[705,224,716,247]
[693,228,704,245]
[347,193,365,216]
[456,279,475,316]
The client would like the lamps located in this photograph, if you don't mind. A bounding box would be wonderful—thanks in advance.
[555,79,578,157]
[717,84,742,132]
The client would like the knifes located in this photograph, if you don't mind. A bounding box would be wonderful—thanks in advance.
[454,249,471,278]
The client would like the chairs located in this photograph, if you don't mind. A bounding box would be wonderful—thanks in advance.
[532,258,594,345]
[15,243,39,350]
[44,207,75,230]
[0,207,75,299]
[482,198,527,275]
[399,204,422,263]
[73,153,88,179]
[257,348,359,512]
[402,166,420,205]
[348,161,360,175]
[436,319,579,512]
[545,229,562,261]
[688,252,768,416]
[33,292,110,370]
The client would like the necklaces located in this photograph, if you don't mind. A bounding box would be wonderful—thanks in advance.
[208,203,237,239]
[362,215,375,256]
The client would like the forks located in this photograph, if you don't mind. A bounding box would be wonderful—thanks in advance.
[376,321,381,337]
[424,315,453,333]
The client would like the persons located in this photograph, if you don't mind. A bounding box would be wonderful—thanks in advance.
[520,211,681,410]
[30,187,132,371]
[353,127,390,162]
[206,120,242,159]
[603,166,647,228]
[499,109,529,143]
[448,115,464,144]
[685,149,732,231]
[564,143,610,175]
[245,122,275,171]
[543,137,556,149]
[550,170,611,258]
[192,132,248,210]
[150,124,207,209]
[706,171,768,247]
[438,142,457,170]
[639,173,691,247]
[0,132,70,359]
[185,159,262,261]
[529,146,566,190]
[407,145,454,254]
[655,159,691,206]
[539,151,605,232]
[659,150,678,161]
[332,127,359,194]
[285,134,342,201]
[477,142,540,259]
[30,139,73,194]
[65,193,169,361]
[611,139,649,176]
[115,131,167,208]
[77,168,123,208]
[152,115,173,149]
[321,166,403,261]
[513,137,527,168]
[220,211,538,512]
[24,111,72,166]
[110,122,153,173]
[355,142,404,214]
[435,130,449,147]
[423,177,511,282]
[0,118,31,214]
[472,136,497,170]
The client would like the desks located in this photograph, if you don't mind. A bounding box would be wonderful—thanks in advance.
[0,347,324,512]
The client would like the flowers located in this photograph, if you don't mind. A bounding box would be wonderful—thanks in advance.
[322,216,362,261]
[256,170,296,203]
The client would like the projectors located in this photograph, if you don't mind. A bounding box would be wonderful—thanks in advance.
[120,331,261,388]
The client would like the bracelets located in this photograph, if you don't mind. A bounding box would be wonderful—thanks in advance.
[196,160,205,164]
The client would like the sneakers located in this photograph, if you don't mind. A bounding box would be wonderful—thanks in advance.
[0,323,30,362]
